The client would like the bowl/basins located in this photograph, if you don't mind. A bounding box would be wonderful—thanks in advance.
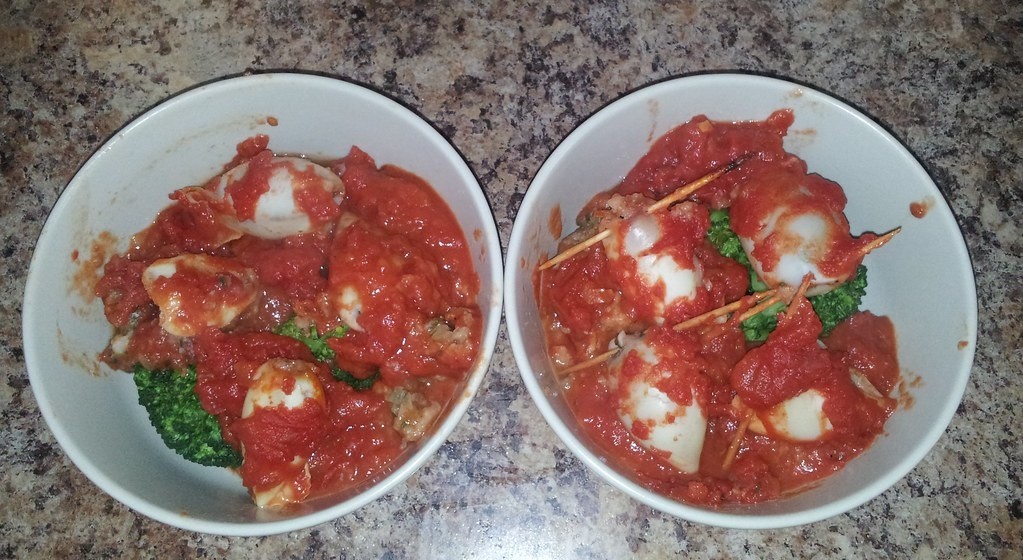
[20,68,502,540]
[505,70,983,529]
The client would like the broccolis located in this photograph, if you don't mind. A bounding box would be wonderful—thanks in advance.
[132,319,382,469]
[705,209,869,344]
